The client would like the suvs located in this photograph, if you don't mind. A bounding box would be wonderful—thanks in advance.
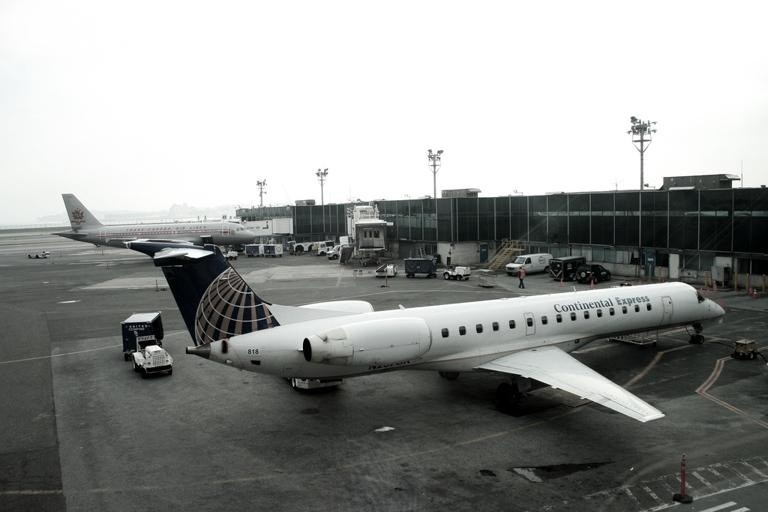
[575,264,611,284]
[443,265,472,280]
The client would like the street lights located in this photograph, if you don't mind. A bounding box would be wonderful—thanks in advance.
[315,167,329,233]
[428,149,443,199]
[627,116,657,191]
[256,179,267,207]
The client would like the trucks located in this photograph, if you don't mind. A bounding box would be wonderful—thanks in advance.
[287,241,341,260]
[120,311,174,380]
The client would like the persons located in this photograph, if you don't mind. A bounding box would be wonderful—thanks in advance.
[518,268,526,288]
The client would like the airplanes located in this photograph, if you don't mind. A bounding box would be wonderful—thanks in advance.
[129,242,725,423]
[49,193,256,249]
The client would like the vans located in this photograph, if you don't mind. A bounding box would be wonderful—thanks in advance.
[505,253,554,276]
